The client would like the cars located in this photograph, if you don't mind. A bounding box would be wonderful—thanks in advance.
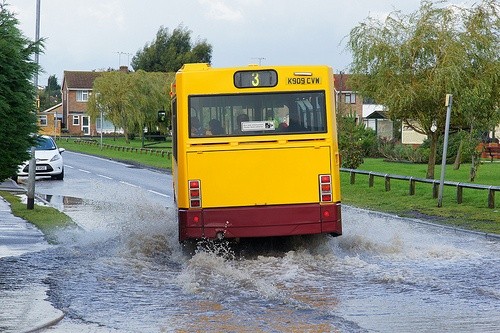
[17,135,65,182]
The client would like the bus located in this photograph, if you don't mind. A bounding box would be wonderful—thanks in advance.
[170,61,342,249]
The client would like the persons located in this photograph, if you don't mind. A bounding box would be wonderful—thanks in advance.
[277,122,288,129]
[190,116,206,136]
[234,113,253,134]
[209,119,226,135]
